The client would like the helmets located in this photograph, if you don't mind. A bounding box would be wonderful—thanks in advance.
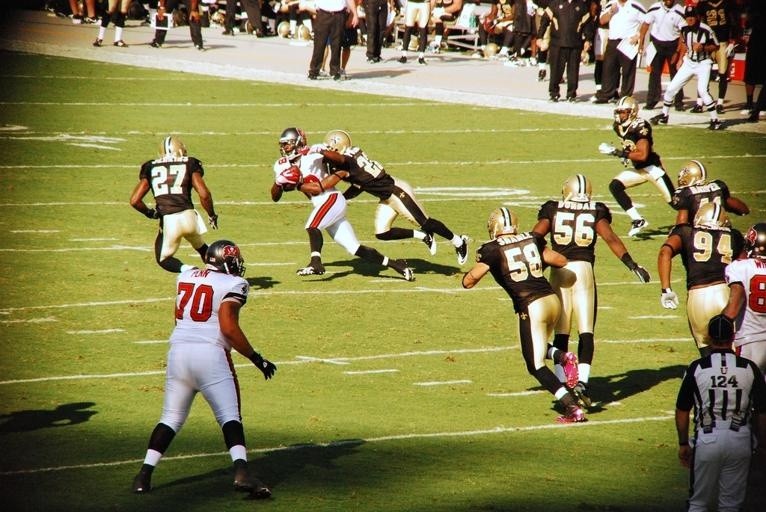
[679,160,707,186]
[279,128,307,160]
[324,129,352,154]
[204,240,246,277]
[744,224,766,255]
[694,203,731,229]
[614,97,638,124]
[562,175,592,202]
[159,136,188,157]
[488,208,520,241]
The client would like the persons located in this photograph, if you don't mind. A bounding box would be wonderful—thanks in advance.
[675,312,766,511]
[129,137,218,271]
[721,223,766,371]
[666,159,750,229]
[461,204,590,422]
[729,11,752,54]
[133,240,277,500]
[37,0,552,83]
[655,201,745,359]
[690,1,738,115]
[310,128,468,264]
[741,2,765,118]
[535,0,589,106]
[270,126,416,285]
[651,5,722,134]
[586,0,610,104]
[589,0,648,106]
[597,95,679,239]
[531,173,650,408]
[637,1,691,113]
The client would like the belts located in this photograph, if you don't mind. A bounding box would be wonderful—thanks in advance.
[608,37,624,43]
[318,8,348,15]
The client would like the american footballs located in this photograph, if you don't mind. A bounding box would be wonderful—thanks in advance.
[280,164,303,192]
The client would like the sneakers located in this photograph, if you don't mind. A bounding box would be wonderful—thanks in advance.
[133,471,152,492]
[714,103,725,114]
[643,102,658,110]
[690,103,703,113]
[456,235,468,265]
[560,351,579,390]
[706,118,721,130]
[573,381,591,408]
[151,41,160,47]
[650,114,669,124]
[400,56,407,63]
[308,72,318,80]
[743,108,760,123]
[233,460,271,498]
[628,216,650,236]
[397,258,416,281]
[555,405,584,423]
[319,69,326,77]
[333,72,341,80]
[422,229,436,255]
[676,103,686,111]
[418,56,425,63]
[93,38,102,45]
[296,264,326,276]
[194,43,203,49]
[114,41,125,45]
[340,68,346,76]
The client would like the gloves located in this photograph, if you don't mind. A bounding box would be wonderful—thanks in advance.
[599,143,615,156]
[301,176,324,199]
[276,166,300,187]
[209,215,219,230]
[661,289,679,309]
[621,253,651,283]
[251,352,276,380]
[149,207,158,219]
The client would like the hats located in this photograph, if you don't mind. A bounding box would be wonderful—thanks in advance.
[685,7,697,17]
[709,314,735,340]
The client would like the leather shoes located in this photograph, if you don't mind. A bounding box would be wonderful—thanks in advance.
[366,55,382,63]
[553,95,559,102]
[567,97,575,102]
